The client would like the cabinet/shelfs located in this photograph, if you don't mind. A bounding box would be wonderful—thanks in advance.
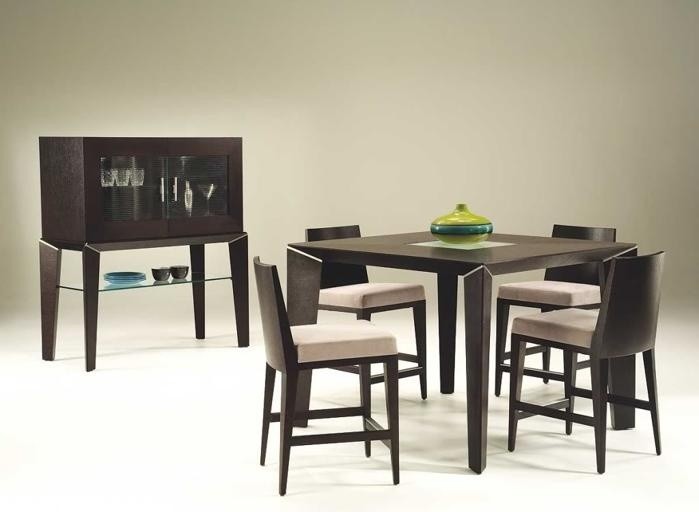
[38,137,249,371]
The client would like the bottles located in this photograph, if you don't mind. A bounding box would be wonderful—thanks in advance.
[183,179,194,219]
[169,176,181,220]
[157,177,167,220]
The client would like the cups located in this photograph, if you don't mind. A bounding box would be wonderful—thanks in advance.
[130,168,145,186]
[115,169,130,186]
[100,168,115,188]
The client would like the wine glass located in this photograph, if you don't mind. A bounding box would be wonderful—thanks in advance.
[197,184,219,217]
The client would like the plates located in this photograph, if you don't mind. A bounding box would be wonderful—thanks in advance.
[103,271,146,284]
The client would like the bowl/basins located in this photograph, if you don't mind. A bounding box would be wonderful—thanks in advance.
[151,269,171,281]
[429,204,494,248]
[170,266,189,279]
